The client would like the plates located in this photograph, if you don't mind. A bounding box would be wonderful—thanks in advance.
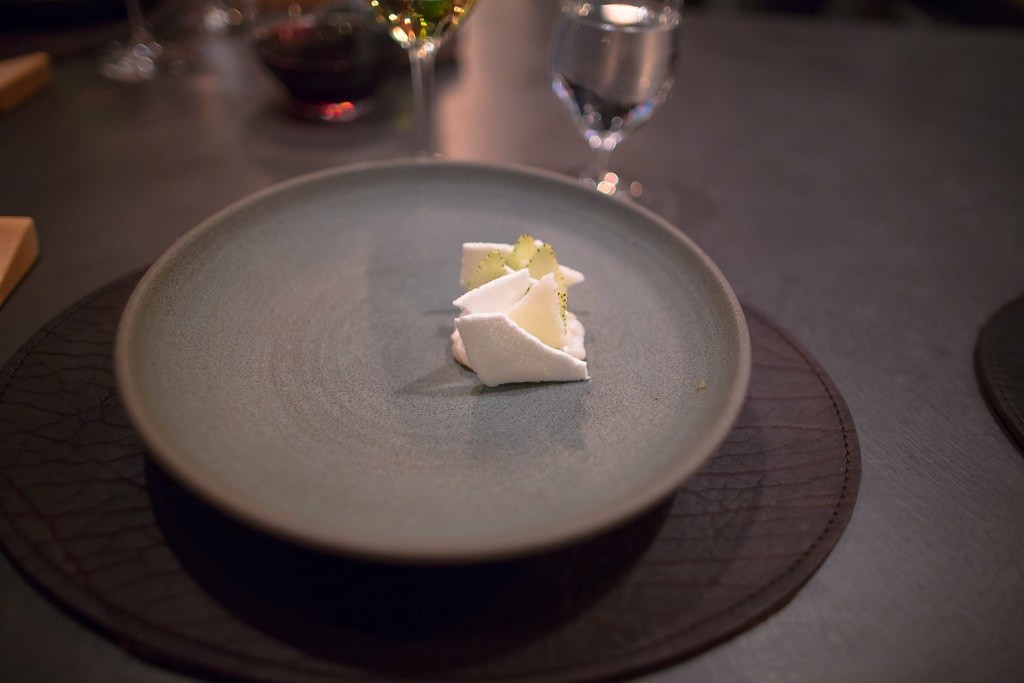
[112,160,751,565]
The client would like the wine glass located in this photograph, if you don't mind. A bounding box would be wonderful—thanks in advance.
[364,0,479,157]
[101,0,187,83]
[544,0,681,220]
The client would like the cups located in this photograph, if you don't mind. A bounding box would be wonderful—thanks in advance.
[252,0,382,120]
